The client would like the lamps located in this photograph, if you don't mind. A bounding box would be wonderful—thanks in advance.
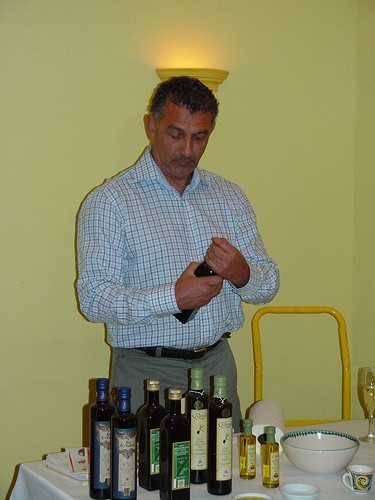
[155,68,229,93]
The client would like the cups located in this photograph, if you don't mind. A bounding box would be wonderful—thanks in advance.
[342,465,374,494]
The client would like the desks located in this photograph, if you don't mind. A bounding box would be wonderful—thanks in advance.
[8,419,375,500]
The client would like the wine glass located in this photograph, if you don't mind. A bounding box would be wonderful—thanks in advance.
[359,367,375,443]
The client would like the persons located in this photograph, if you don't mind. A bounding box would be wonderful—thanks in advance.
[77,77,279,432]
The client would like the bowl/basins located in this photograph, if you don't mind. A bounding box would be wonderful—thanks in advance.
[280,430,360,474]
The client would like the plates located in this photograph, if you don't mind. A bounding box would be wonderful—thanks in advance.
[280,482,321,500]
[233,492,272,500]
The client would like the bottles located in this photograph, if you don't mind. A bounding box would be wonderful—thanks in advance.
[173,261,218,324]
[160,387,190,500]
[261,426,280,488]
[90,378,114,500]
[110,387,138,500]
[207,375,232,496]
[239,419,256,481]
[138,378,166,491]
[186,368,209,484]
